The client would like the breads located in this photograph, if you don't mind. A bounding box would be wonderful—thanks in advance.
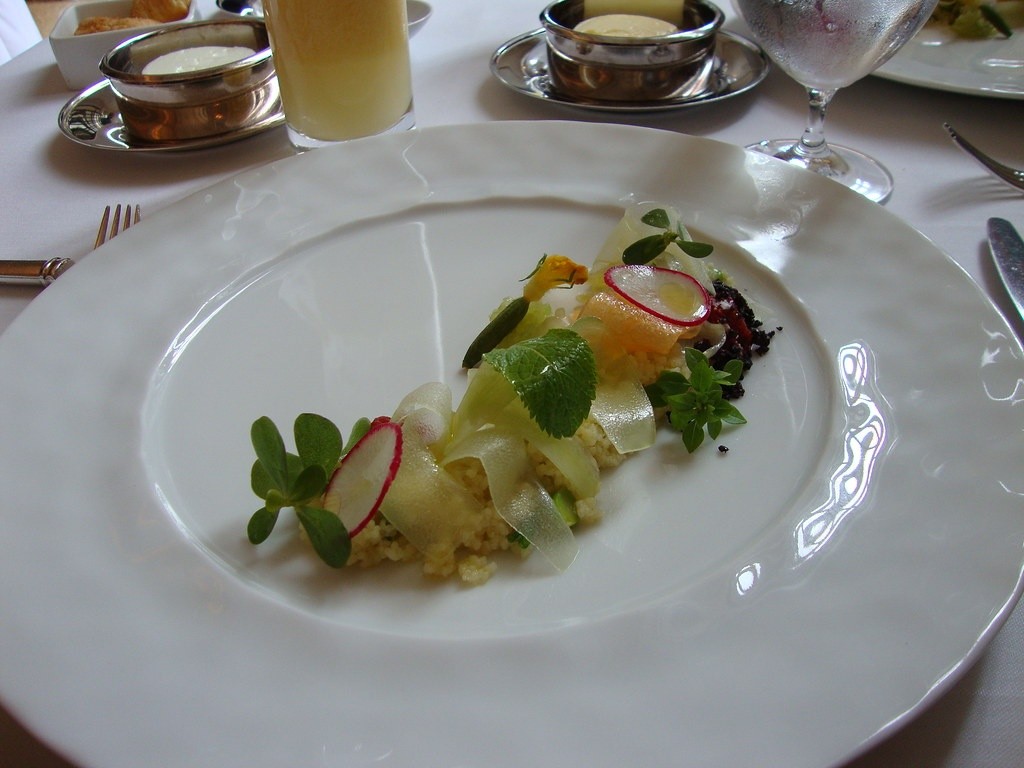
[73,0,193,37]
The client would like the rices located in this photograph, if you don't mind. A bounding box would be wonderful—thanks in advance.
[291,413,638,584]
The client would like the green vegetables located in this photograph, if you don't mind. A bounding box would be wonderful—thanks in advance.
[246,209,749,566]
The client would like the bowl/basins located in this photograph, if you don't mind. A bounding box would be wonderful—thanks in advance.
[49,0,196,91]
[537,0,727,104]
[406,0,432,39]
[98,19,275,142]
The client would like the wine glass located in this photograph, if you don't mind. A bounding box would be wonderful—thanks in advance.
[731,0,943,204]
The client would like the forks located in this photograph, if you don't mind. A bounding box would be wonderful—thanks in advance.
[94,204,140,250]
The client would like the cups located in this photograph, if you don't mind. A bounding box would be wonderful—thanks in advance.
[261,0,417,155]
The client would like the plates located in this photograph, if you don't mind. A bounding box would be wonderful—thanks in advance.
[869,28,1024,100]
[0,121,1024,766]
[216,0,263,18]
[56,72,288,154]
[490,26,771,114]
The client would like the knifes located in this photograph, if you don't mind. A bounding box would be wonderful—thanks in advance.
[987,217,1024,322]
